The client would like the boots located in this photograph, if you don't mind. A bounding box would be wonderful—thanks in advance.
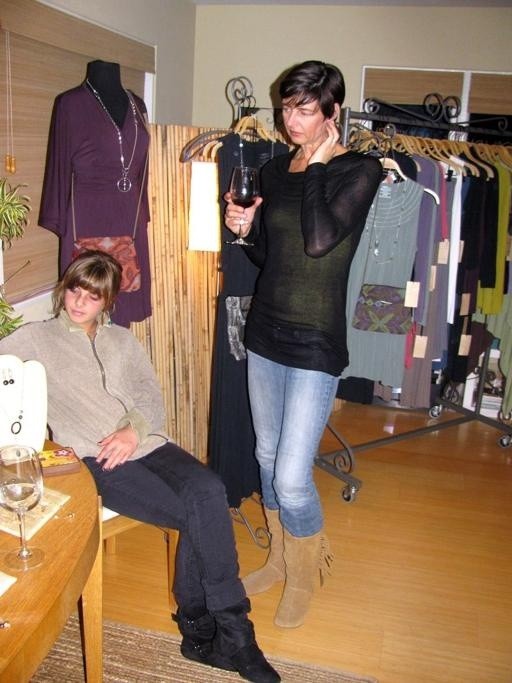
[276,530,334,629]
[171,605,237,672]
[242,507,286,596]
[210,598,281,683]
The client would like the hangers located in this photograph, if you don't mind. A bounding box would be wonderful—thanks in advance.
[199,94,289,162]
[347,110,512,180]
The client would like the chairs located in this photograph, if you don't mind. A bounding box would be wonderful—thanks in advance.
[45,424,182,616]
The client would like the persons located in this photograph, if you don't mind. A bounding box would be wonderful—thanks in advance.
[224,59,384,630]
[37,59,152,330]
[1,249,283,683]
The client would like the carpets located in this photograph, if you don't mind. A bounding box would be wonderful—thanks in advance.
[27,612,383,682]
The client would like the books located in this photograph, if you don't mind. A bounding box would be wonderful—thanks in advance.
[1,465,72,542]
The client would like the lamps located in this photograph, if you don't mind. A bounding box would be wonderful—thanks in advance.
[183,159,225,255]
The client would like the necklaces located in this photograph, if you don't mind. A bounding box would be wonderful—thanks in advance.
[372,178,401,256]
[238,135,274,179]
[86,78,139,195]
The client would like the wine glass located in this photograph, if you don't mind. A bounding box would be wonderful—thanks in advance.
[226,167,262,245]
[1,446,51,573]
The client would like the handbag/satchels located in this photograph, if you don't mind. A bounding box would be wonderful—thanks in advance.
[72,236,142,294]
[352,285,412,334]
[225,294,255,361]
